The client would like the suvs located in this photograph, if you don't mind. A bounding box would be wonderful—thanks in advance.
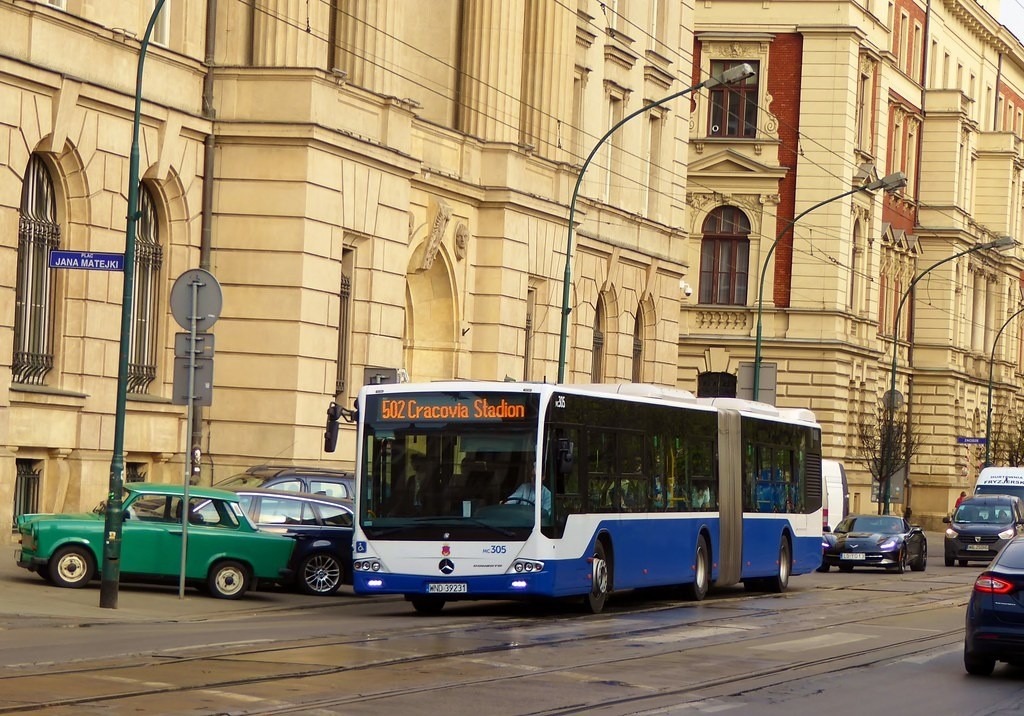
[942,493,1024,567]
[130,463,356,520]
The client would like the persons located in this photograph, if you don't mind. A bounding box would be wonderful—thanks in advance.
[952,491,967,515]
[608,471,711,509]
[500,468,551,517]
[406,454,432,516]
[997,510,1011,523]
[890,521,901,531]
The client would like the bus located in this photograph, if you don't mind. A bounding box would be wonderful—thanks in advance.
[322,376,828,618]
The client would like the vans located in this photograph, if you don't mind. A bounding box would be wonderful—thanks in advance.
[973,466,1024,521]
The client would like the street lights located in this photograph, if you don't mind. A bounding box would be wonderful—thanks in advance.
[882,233,1017,515]
[558,62,757,383]
[753,171,907,402]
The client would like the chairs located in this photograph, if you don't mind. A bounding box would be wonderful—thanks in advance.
[174,500,183,524]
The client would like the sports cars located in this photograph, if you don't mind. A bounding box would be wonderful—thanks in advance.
[816,514,928,575]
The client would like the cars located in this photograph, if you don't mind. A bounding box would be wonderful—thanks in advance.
[963,534,1024,676]
[136,488,356,598]
[14,481,298,601]
